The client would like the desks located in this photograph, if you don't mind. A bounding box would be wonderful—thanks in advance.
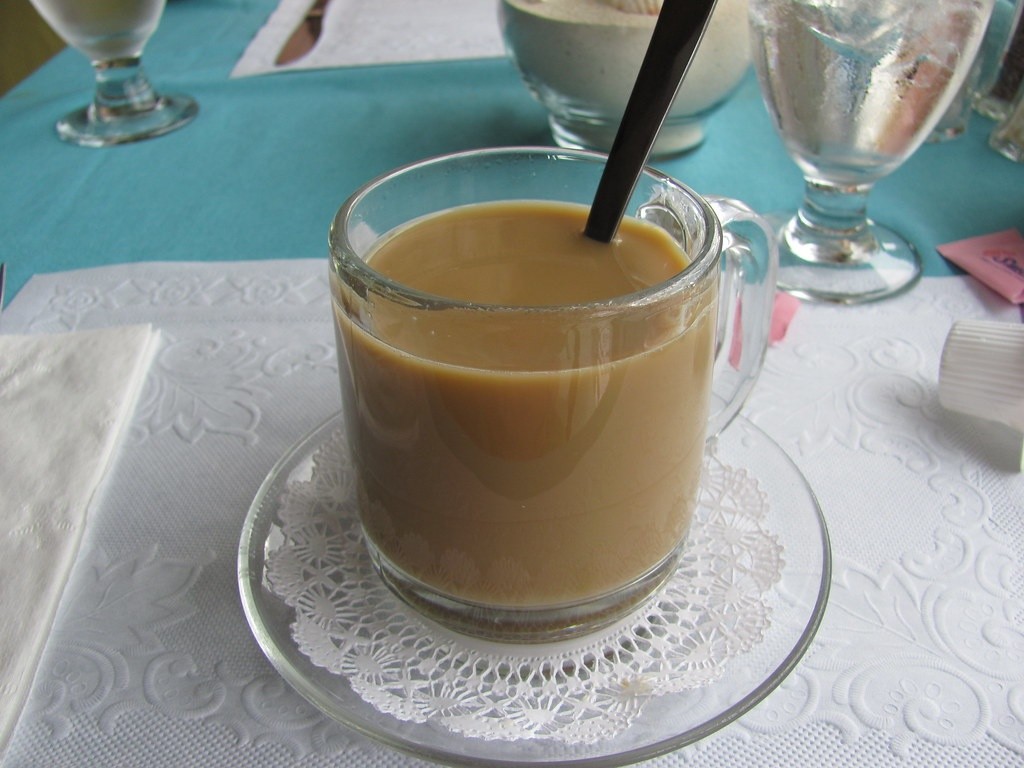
[0,0,1024,768]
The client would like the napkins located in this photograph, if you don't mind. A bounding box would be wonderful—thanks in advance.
[0,323,168,756]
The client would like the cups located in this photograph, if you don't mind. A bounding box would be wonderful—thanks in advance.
[939,320,1023,435]
[498,0,753,158]
[328,146,779,645]
[921,0,1024,164]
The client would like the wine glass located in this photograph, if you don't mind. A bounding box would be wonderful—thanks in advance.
[749,0,995,308]
[26,0,198,148]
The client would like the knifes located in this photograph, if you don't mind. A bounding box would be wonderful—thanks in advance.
[275,0,327,65]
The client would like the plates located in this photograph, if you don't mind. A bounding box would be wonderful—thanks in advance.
[238,391,831,767]
